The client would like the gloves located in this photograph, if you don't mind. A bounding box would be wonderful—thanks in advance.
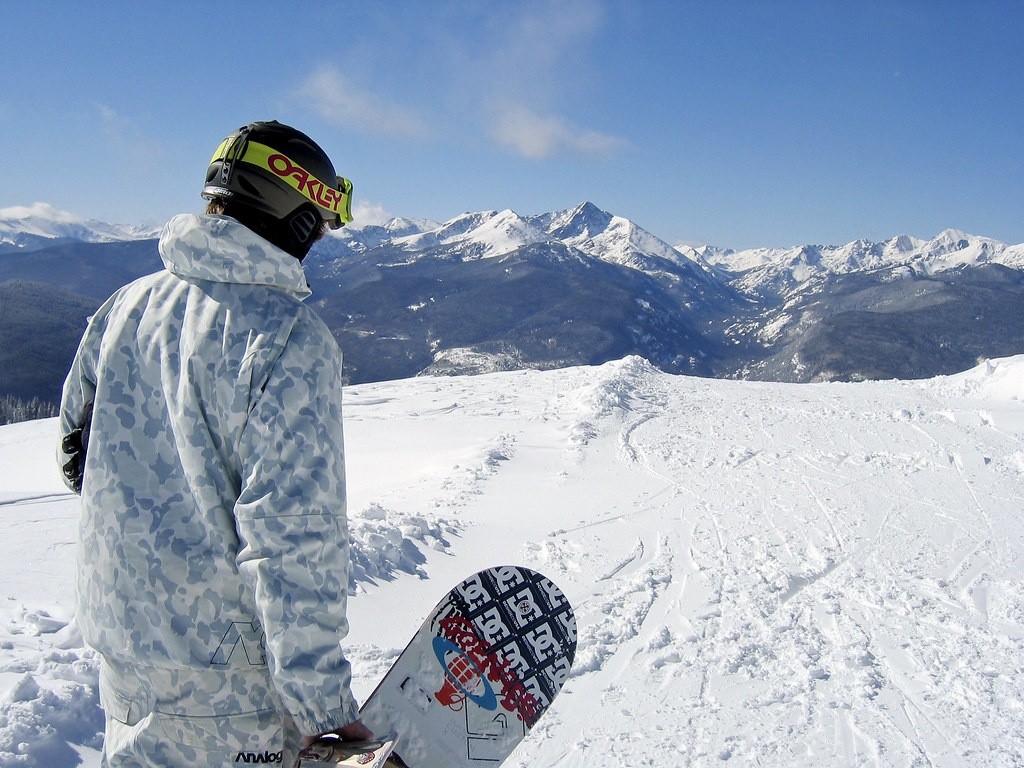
[62,403,93,492]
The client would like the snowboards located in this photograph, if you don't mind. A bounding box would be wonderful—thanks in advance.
[296,562,582,768]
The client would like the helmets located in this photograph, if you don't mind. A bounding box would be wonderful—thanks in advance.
[201,120,337,222]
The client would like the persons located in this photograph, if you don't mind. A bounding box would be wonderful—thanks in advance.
[53,121,380,766]
